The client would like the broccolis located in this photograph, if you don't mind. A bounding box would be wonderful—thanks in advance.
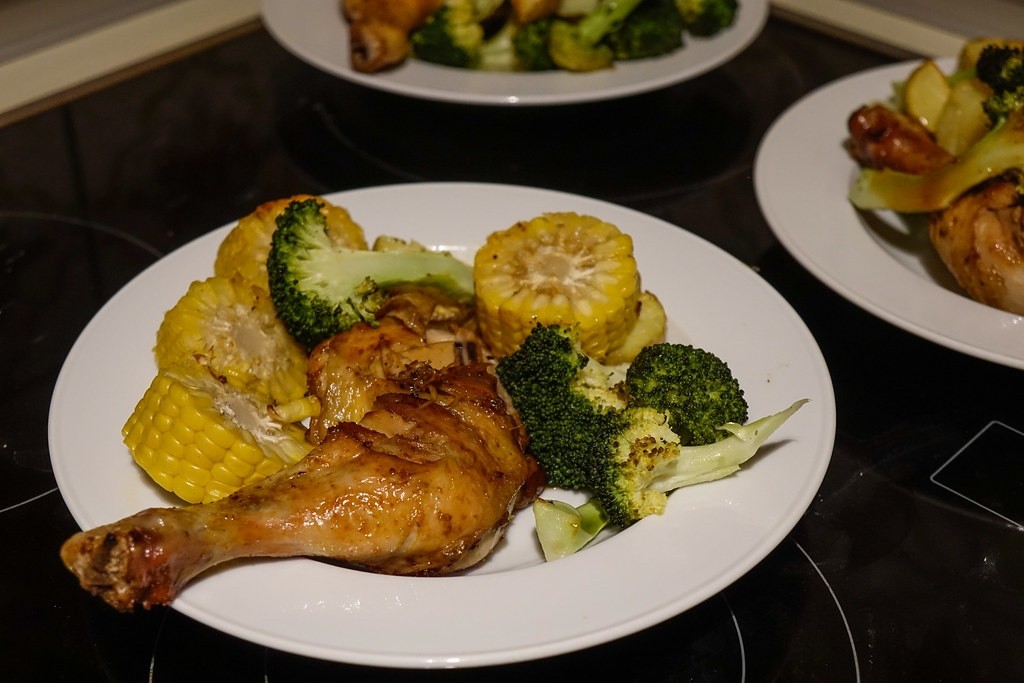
[850,45,1024,215]
[410,0,738,73]
[267,198,474,353]
[495,321,810,563]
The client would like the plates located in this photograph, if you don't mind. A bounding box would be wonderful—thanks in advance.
[256,1,773,105]
[754,54,1023,385]
[47,178,837,675]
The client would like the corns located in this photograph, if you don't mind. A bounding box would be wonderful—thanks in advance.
[474,211,642,365]
[122,194,368,502]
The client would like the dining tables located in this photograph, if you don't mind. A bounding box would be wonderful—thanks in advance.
[0,0,1024,683]
